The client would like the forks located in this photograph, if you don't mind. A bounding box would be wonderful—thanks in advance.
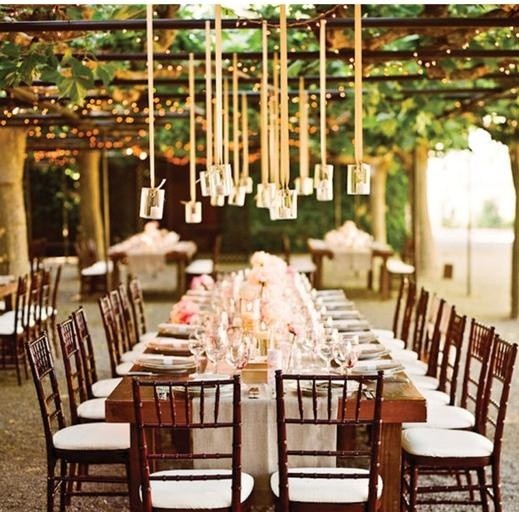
[152,384,171,401]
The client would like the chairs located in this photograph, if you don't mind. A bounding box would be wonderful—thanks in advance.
[128,372,255,511]
[95,273,148,379]
[0,219,519,511]
[23,328,131,511]
[399,316,496,501]
[407,305,468,406]
[271,367,384,512]
[406,298,454,389]
[53,314,102,492]
[71,304,124,400]
[400,333,519,511]
[0,256,64,387]
[370,273,432,377]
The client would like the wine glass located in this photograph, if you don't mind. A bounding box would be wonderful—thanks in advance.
[189,267,361,396]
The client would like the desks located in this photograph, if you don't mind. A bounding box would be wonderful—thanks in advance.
[104,288,428,511]
[78,219,419,302]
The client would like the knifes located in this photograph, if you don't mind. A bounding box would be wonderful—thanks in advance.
[247,387,260,399]
[358,382,378,399]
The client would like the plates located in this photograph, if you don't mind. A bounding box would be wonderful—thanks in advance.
[291,372,368,398]
[172,374,237,395]
[334,321,406,378]
[132,323,200,375]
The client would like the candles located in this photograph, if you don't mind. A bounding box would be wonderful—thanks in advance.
[150,204,160,217]
[355,180,368,194]
